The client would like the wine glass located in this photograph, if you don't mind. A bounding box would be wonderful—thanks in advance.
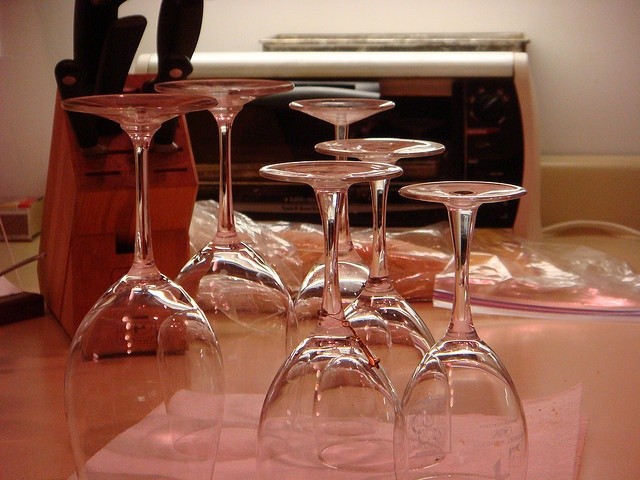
[157,78,297,460]
[60,94,227,479]
[258,160,401,480]
[392,180,528,479]
[285,99,395,419]
[312,137,449,474]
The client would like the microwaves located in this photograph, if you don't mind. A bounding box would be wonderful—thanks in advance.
[126,53,543,243]
